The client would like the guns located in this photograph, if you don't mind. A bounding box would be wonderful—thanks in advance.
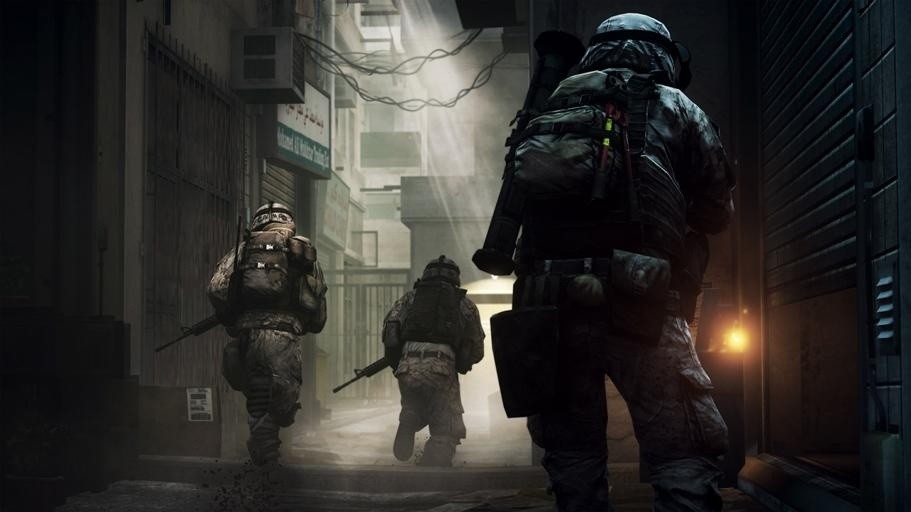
[153,314,222,351]
[333,357,390,393]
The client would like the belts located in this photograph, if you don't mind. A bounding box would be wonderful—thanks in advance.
[403,350,448,362]
[521,255,614,282]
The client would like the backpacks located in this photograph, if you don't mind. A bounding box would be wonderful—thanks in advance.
[232,236,296,312]
[512,67,656,247]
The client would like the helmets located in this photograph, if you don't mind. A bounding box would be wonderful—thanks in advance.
[248,199,295,233]
[577,11,684,85]
[420,255,462,287]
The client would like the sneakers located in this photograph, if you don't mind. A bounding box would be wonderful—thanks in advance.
[392,421,416,462]
[246,362,279,418]
